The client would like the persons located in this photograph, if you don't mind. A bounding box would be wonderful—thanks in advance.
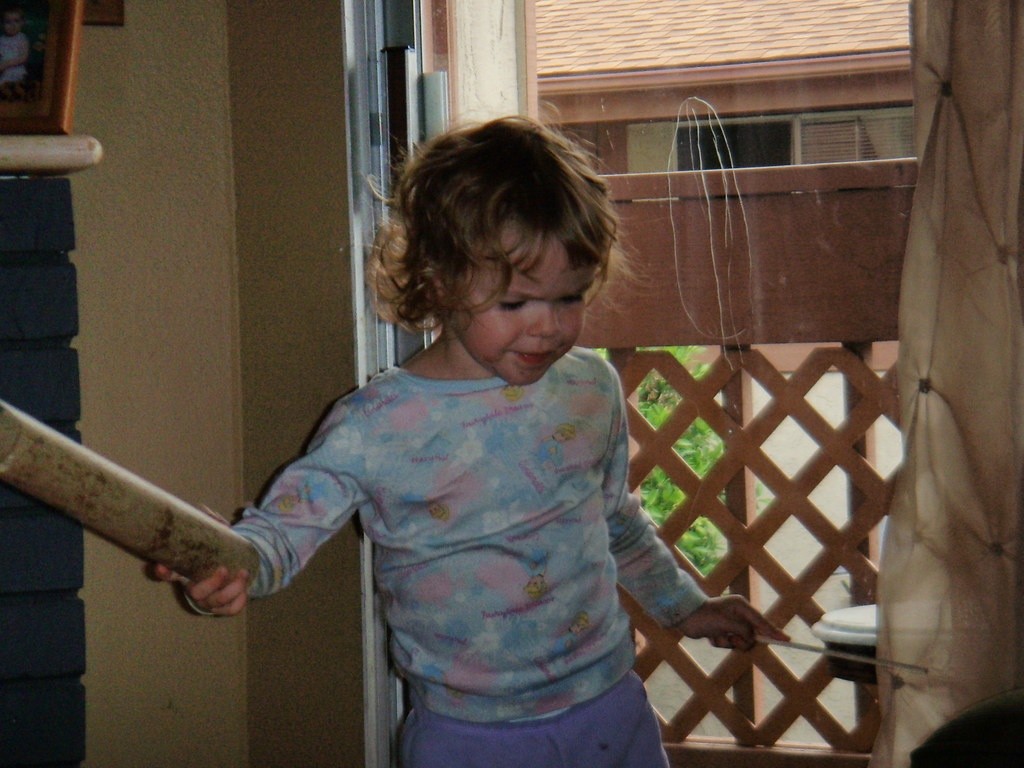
[153,118,791,768]
[0,7,32,102]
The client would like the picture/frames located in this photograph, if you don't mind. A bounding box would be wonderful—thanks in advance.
[0,0,83,135]
